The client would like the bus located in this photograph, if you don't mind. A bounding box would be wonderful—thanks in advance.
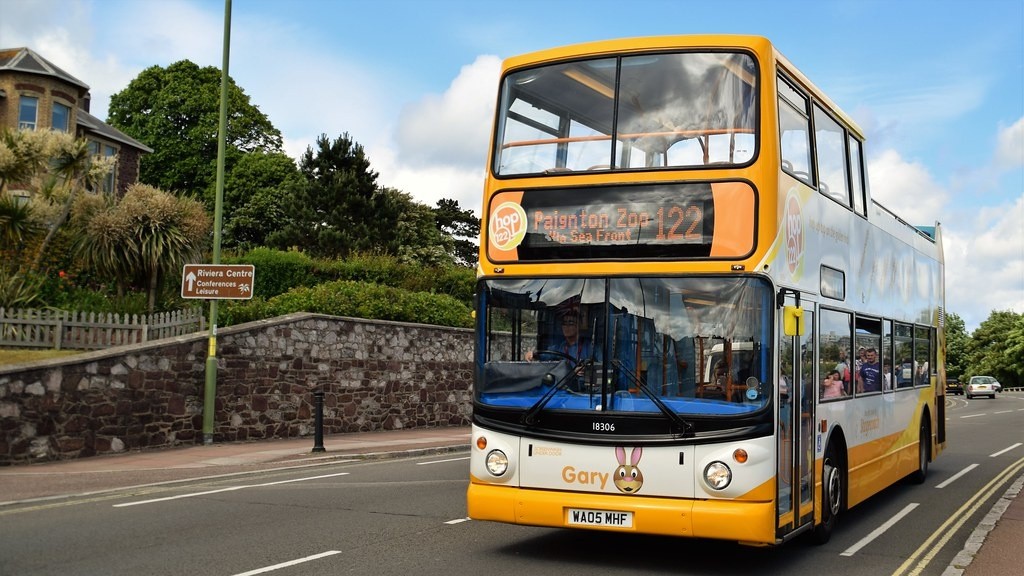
[468,33,947,543]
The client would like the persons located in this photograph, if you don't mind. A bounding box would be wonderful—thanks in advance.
[524,310,602,393]
[696,359,739,403]
[822,346,929,399]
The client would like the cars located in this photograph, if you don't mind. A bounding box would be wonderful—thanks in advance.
[946,378,964,395]
[966,376,995,399]
[980,377,1002,393]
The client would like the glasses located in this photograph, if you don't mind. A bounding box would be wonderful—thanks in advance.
[562,321,578,326]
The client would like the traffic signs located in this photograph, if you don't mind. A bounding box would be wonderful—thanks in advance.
[181,264,255,300]
